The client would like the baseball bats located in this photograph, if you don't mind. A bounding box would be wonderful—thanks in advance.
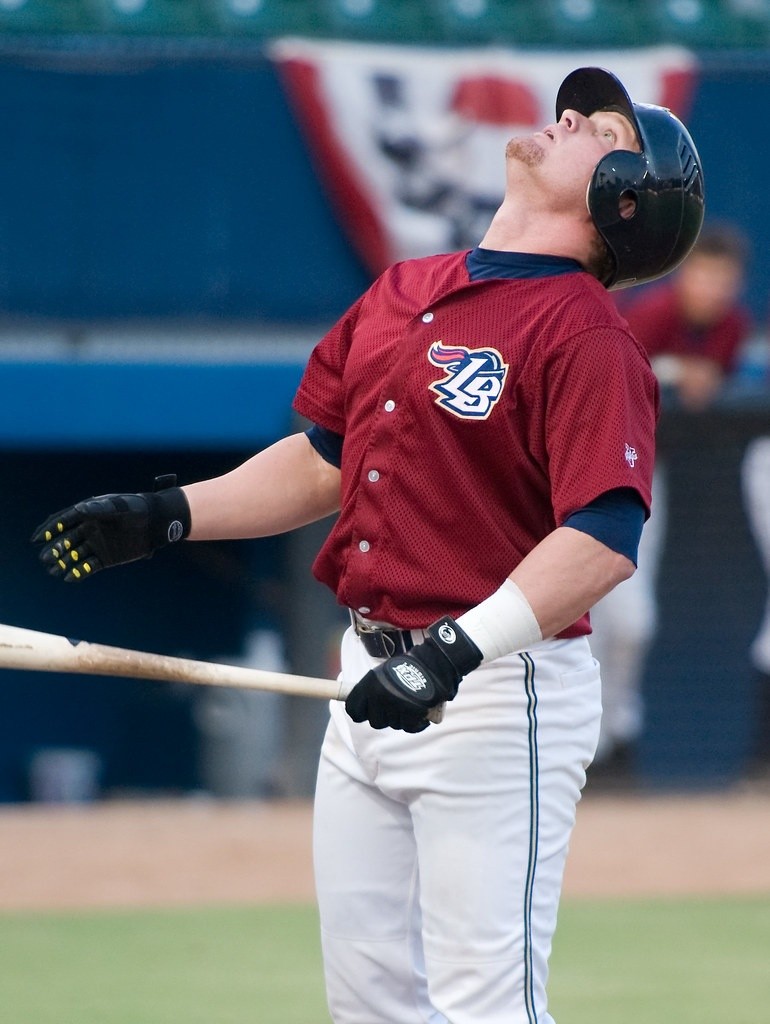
[1,619,452,725]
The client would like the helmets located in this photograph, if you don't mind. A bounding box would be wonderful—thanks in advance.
[555,66,706,292]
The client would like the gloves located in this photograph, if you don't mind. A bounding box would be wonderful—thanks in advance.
[342,615,483,733]
[29,473,191,584]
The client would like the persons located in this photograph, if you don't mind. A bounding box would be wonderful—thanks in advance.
[625,235,749,407]
[30,68,705,1024]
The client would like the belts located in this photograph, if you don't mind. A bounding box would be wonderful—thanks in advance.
[343,606,413,660]
